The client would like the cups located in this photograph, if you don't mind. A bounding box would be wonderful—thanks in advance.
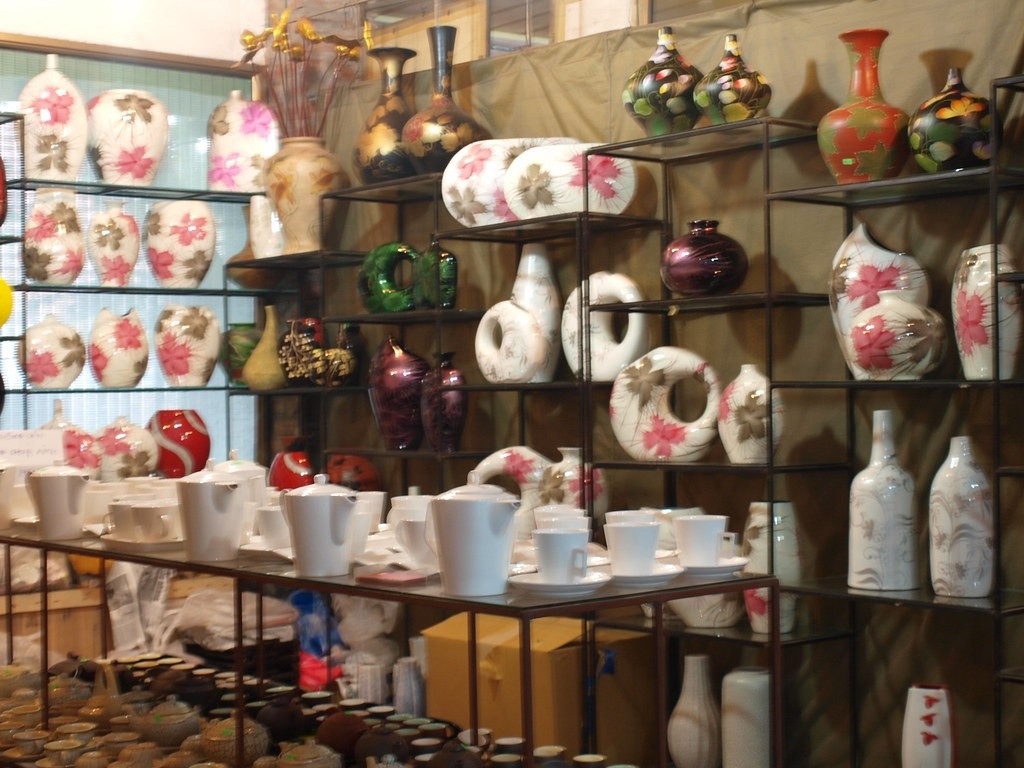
[532,501,737,581]
[1,652,642,768]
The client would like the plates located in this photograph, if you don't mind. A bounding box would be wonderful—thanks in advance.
[507,547,749,598]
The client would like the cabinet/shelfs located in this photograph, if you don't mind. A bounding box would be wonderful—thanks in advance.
[0,73,1024,768]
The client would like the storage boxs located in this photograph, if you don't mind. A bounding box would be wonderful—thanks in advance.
[421,610,656,763]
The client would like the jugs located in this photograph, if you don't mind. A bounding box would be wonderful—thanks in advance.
[24,450,526,598]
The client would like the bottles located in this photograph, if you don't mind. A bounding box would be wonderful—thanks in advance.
[723,667,770,768]
[901,683,956,768]
[744,501,802,635]
[664,655,722,768]
[928,433,1000,595]
[1,20,1023,515]
[845,408,923,591]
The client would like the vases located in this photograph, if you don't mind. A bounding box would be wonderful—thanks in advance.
[10,25,1021,768]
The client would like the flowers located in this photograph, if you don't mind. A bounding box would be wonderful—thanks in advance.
[228,4,374,138]
